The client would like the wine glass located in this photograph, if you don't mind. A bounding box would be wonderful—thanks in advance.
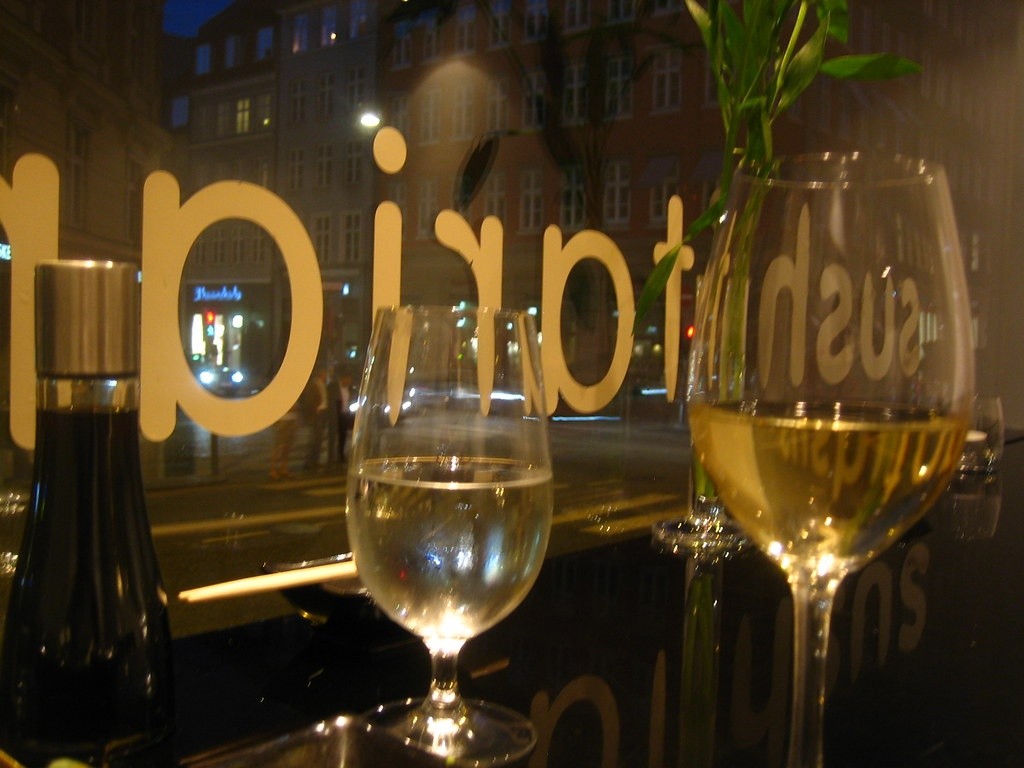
[341,303,553,767]
[687,144,977,768]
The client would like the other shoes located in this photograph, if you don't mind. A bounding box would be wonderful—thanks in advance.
[270,469,280,479]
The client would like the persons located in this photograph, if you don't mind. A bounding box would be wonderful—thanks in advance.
[305,365,359,480]
[268,402,302,479]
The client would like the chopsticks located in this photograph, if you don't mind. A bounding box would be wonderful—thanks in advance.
[175,483,693,616]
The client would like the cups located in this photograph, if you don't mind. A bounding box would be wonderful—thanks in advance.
[956,394,1006,474]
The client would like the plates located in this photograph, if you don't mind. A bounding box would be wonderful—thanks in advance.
[257,549,373,614]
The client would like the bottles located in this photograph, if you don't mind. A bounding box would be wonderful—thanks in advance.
[0,257,185,768]
